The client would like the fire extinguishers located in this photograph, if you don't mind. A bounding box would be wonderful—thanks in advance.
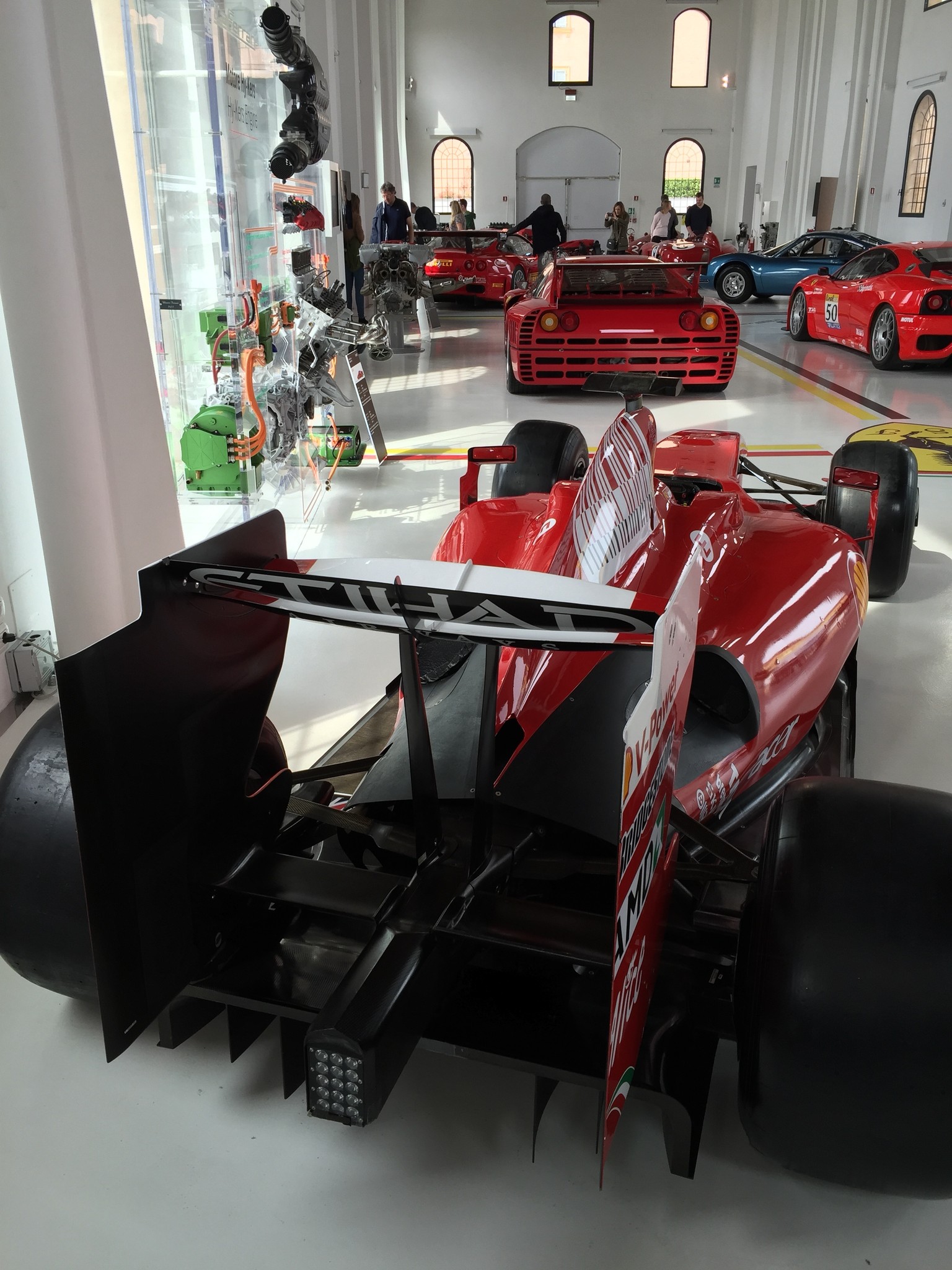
[626,228,635,244]
[748,236,756,252]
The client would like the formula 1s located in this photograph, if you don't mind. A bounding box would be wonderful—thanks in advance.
[0,390,951,1197]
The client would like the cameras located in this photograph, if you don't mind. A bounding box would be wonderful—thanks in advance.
[607,213,612,216]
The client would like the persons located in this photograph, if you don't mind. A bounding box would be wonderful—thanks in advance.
[449,201,466,248]
[411,201,437,231]
[343,191,372,324]
[681,206,692,238]
[370,181,414,246]
[501,194,567,277]
[445,222,449,231]
[685,192,713,237]
[604,202,629,255]
[655,194,678,239]
[650,200,672,243]
[458,199,476,230]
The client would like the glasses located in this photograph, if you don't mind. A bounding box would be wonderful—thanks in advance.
[382,193,392,198]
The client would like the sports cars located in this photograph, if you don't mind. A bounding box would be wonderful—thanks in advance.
[424,221,739,310]
[688,229,894,305]
[502,248,740,395]
[785,239,952,370]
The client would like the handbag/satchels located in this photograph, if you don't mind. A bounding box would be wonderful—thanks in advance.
[343,229,362,272]
[606,239,617,250]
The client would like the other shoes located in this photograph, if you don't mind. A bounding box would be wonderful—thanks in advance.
[359,318,369,324]
